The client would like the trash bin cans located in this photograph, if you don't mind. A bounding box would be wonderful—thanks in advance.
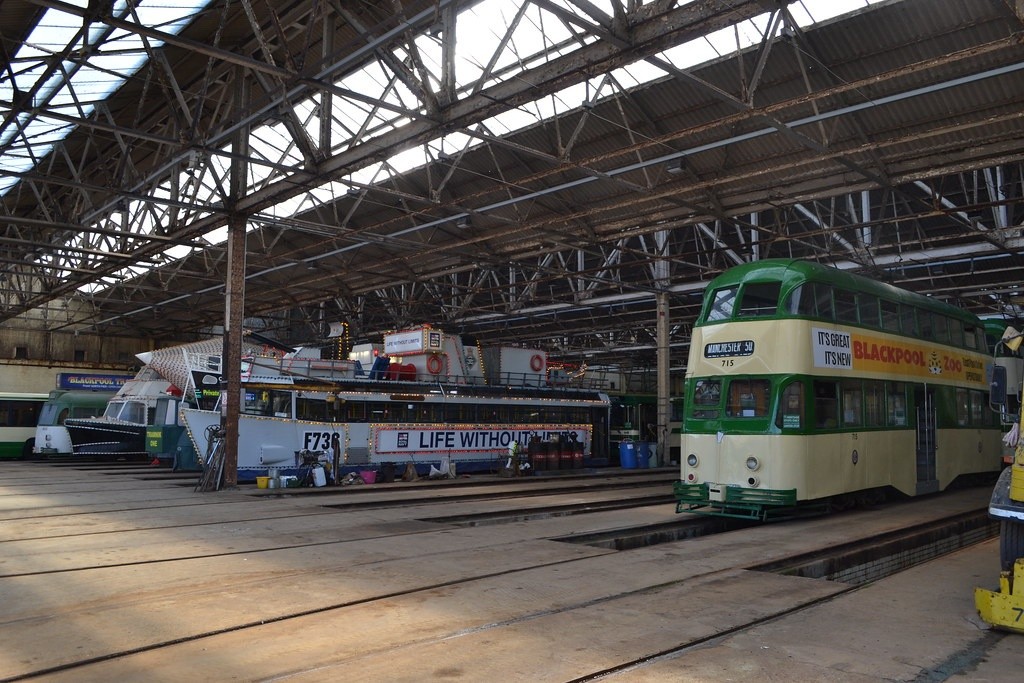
[617,440,657,469]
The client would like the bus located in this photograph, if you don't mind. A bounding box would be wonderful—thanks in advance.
[179,375,604,487]
[65,358,365,458]
[0,392,49,457]
[34,390,126,456]
[670,258,1020,523]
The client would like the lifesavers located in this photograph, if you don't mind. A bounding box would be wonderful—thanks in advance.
[531,355,545,372]
[428,355,444,374]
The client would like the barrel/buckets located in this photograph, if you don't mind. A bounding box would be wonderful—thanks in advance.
[528,442,585,471]
[256,468,280,488]
[359,471,376,484]
[312,467,327,487]
[619,440,658,469]
